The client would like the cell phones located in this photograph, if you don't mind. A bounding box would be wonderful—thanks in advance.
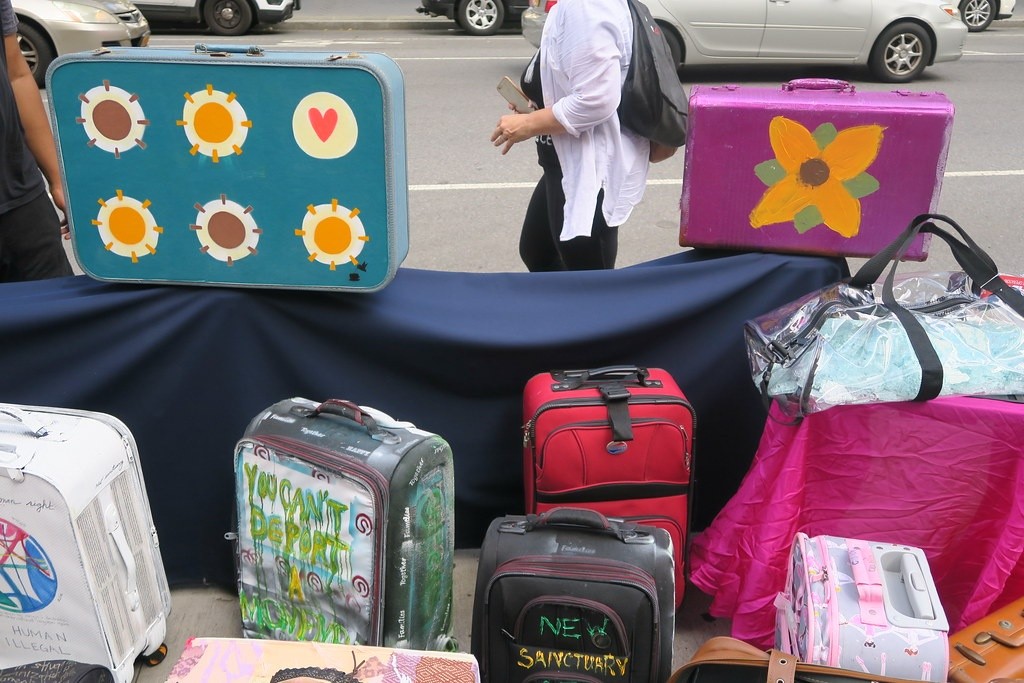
[496,76,539,114]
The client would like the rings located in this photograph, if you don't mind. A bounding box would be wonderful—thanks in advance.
[500,134,505,141]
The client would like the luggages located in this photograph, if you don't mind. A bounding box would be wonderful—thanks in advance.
[775,532,948,683]
[0,402,171,683]
[523,364,696,609]
[231,397,456,652]
[469,507,676,683]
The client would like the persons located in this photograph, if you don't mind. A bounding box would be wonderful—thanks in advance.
[0,0,77,282]
[491,0,651,272]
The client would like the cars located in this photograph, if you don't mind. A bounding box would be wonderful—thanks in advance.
[946,0,1020,32]
[103,0,303,39]
[4,0,152,90]
[515,0,970,82]
[412,1,535,36]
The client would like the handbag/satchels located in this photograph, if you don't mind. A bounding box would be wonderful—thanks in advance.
[619,0,688,148]
[743,213,1024,426]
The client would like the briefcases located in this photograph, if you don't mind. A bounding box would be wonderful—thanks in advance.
[679,78,954,263]
[45,43,408,293]
[948,595,1024,683]
